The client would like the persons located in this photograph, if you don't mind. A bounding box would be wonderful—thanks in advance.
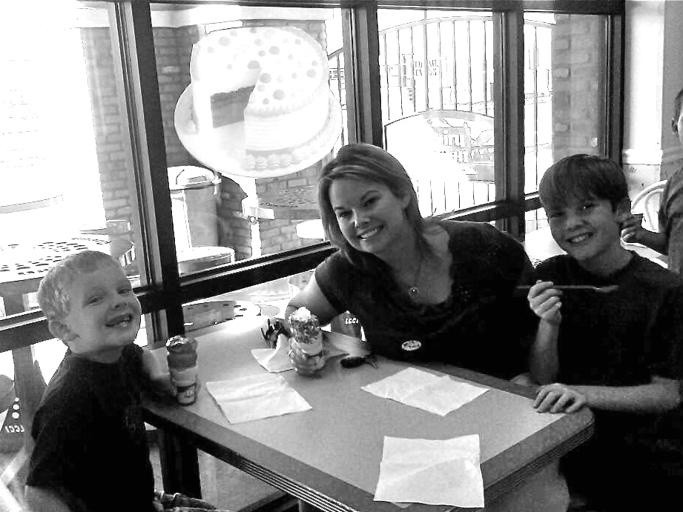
[618,89,682,276]
[18,247,205,511]
[522,152,682,510]
[281,140,537,380]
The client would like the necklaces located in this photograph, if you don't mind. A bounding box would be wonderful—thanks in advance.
[388,255,427,299]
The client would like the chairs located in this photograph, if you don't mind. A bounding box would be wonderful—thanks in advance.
[630,180,667,233]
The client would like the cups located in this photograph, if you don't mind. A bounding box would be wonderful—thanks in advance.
[294,336,325,371]
[165,367,197,405]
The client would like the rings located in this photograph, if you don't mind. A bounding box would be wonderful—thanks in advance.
[288,350,294,357]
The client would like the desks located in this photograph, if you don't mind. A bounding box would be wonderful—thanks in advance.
[0,234,133,488]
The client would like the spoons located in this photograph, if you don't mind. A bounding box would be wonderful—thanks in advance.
[515,284,616,294]
